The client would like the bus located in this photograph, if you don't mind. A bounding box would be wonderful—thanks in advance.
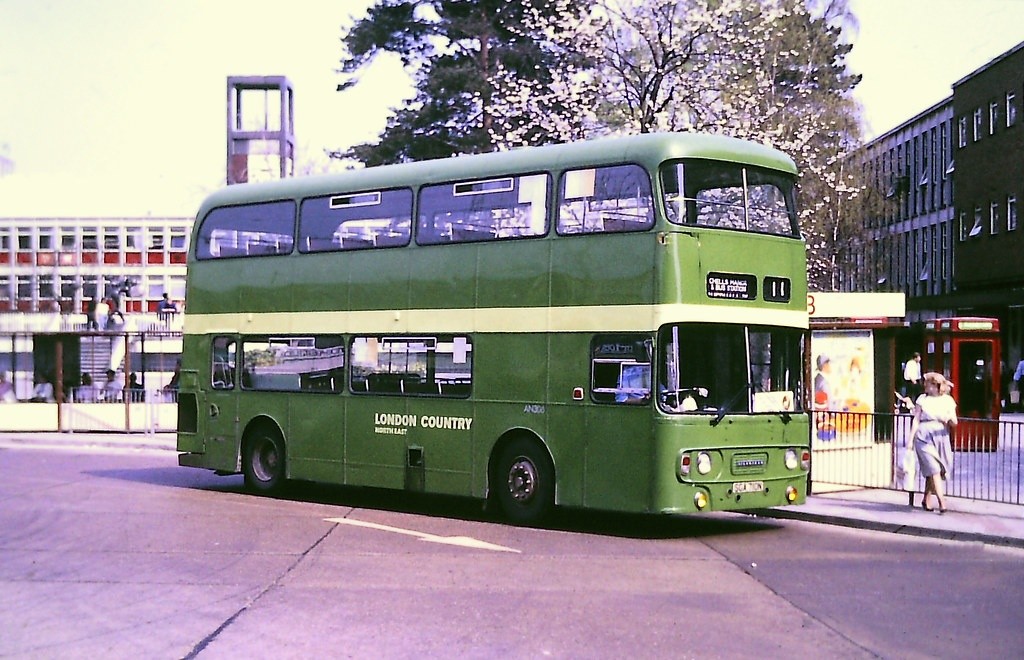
[171,129,816,529]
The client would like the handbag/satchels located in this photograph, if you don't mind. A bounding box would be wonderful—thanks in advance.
[896,448,915,491]
[1011,391,1020,403]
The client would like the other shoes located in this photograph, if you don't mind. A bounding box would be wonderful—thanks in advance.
[938,504,947,512]
[922,501,934,511]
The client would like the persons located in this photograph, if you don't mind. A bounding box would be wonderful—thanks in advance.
[905,370,959,514]
[100,369,122,403]
[616,345,675,405]
[159,292,181,312]
[100,296,129,329]
[903,352,923,410]
[32,368,70,401]
[961,348,1024,425]
[122,373,144,403]
[163,360,182,400]
[84,295,100,332]
[0,375,21,403]
[75,369,98,403]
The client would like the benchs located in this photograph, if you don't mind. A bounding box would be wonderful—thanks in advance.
[219,222,535,258]
[298,366,471,396]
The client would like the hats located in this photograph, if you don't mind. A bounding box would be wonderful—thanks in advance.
[816,355,828,370]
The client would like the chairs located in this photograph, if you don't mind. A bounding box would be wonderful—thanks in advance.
[603,210,652,231]
